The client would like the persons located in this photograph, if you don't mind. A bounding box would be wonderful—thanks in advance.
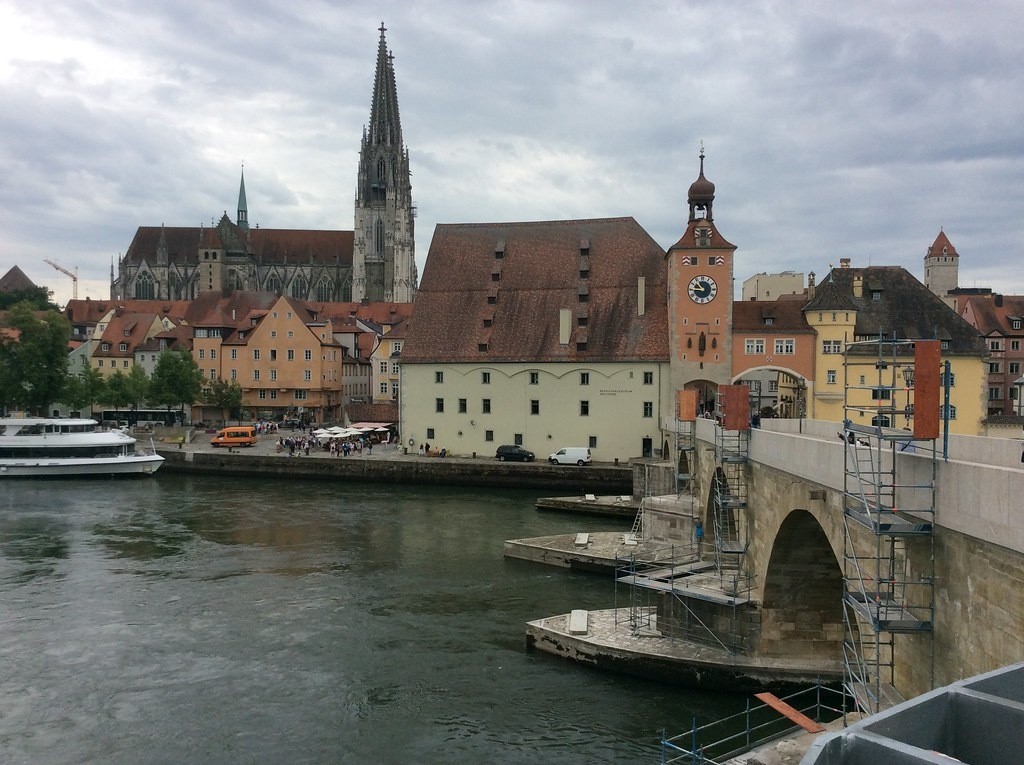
[838,420,853,444]
[749,411,761,429]
[419,443,424,456]
[699,410,710,418]
[441,448,446,457]
[694,522,704,553]
[290,421,312,433]
[276,433,398,457]
[434,446,439,456]
[259,419,281,435]
[897,427,916,452]
[398,443,403,455]
[425,443,430,454]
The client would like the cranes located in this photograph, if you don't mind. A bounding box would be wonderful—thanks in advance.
[43,259,78,299]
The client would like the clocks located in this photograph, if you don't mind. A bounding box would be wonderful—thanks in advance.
[687,274,718,304]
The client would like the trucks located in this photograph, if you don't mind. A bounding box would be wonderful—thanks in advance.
[549,447,591,466]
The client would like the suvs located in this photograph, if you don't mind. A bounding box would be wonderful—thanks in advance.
[496,445,535,463]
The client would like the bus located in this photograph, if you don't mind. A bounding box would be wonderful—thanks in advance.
[102,410,187,429]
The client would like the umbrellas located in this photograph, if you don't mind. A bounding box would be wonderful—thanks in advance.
[313,426,389,437]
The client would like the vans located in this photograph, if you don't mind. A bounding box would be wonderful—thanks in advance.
[210,427,257,448]
[279,419,301,428]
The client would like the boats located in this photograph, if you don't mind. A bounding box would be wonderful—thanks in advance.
[0,409,166,478]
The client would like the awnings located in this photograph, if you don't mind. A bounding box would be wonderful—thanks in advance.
[347,423,393,428]
